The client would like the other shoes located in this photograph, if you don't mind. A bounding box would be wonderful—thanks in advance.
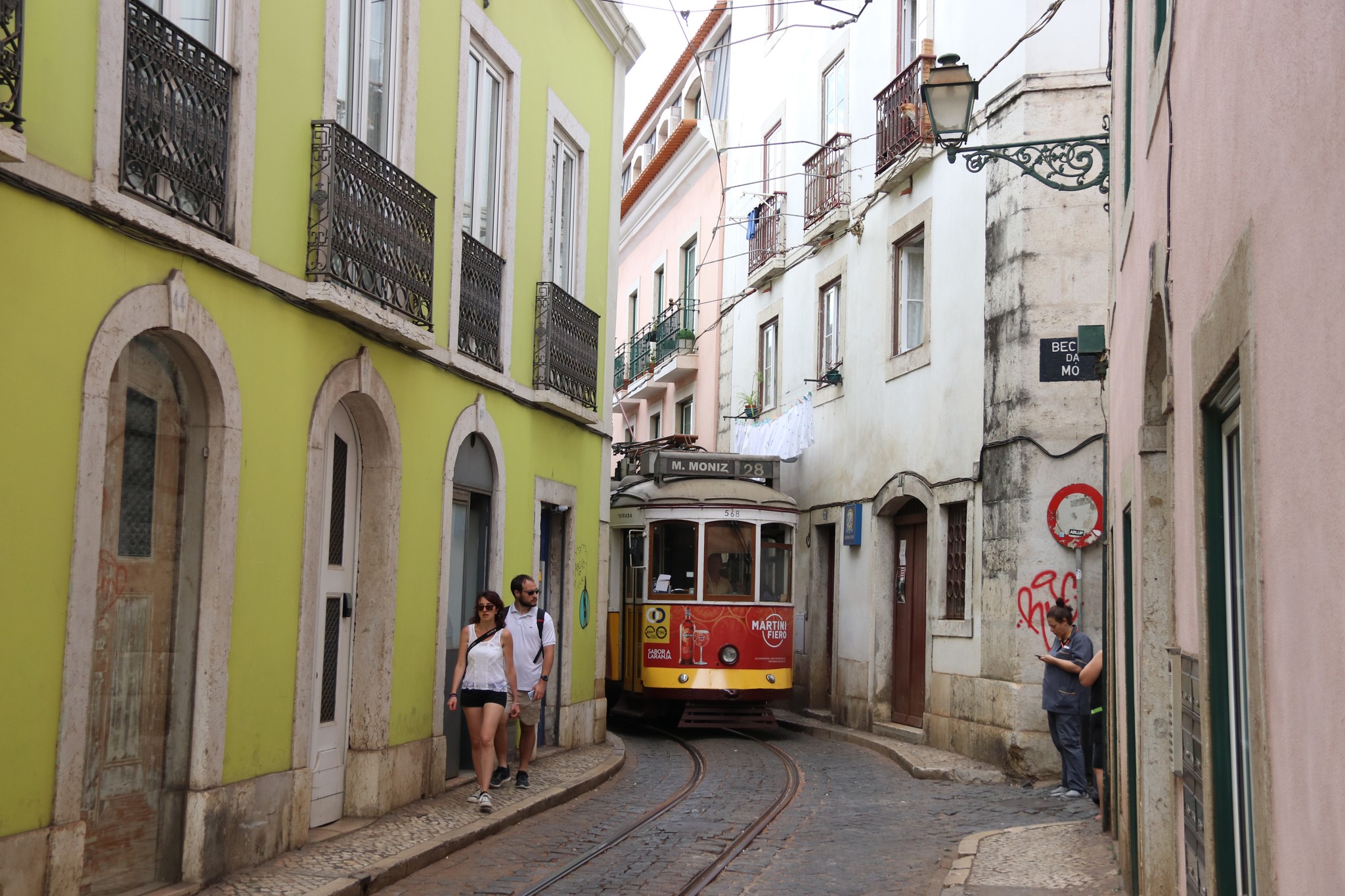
[1060,790,1088,801]
[1047,786,1069,797]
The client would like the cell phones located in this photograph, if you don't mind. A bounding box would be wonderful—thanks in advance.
[1034,654,1041,657]
[528,690,535,701]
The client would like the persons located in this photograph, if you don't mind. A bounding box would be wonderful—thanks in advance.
[488,574,556,788]
[447,591,521,808]
[1041,598,1093,801]
[1079,648,1108,820]
[704,552,738,595]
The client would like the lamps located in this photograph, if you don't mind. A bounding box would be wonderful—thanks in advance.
[915,52,1111,201]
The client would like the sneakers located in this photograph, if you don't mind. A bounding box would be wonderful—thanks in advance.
[479,792,493,808]
[467,785,482,803]
[489,765,511,787]
[514,770,530,789]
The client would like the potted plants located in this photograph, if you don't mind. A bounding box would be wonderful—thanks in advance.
[734,366,765,418]
[613,376,633,393]
[818,360,842,382]
[676,326,696,354]
[648,321,660,342]
[635,339,676,379]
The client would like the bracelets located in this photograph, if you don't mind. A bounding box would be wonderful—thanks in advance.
[513,703,520,705]
[449,693,457,698]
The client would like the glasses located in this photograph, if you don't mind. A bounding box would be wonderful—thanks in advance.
[523,589,539,596]
[475,604,495,611]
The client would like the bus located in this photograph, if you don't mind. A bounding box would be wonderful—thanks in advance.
[606,434,802,733]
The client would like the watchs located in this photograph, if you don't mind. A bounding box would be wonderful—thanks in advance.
[540,675,548,682]
